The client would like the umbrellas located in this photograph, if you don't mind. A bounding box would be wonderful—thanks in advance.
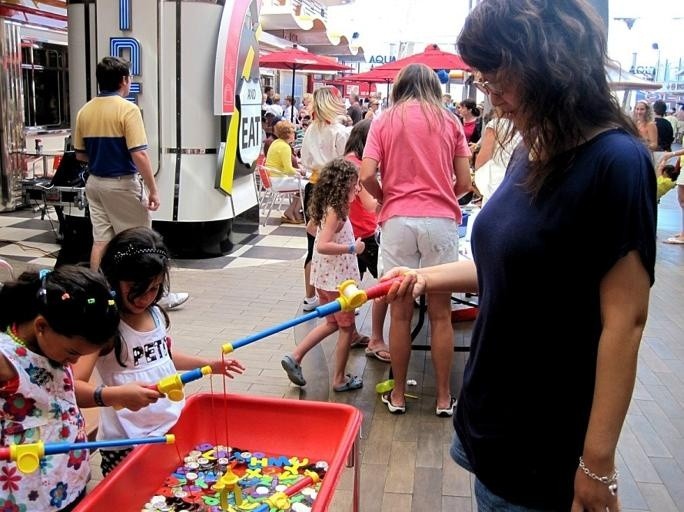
[321,69,401,108]
[369,45,474,72]
[603,61,662,92]
[258,46,353,126]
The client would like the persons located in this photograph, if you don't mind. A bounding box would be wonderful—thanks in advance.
[380,2,656,511]
[0,265,128,512]
[340,119,392,364]
[282,160,365,392]
[75,57,190,310]
[70,225,244,480]
[359,64,473,417]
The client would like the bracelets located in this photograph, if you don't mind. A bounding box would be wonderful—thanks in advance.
[579,457,620,495]
[348,241,356,255]
[92,383,111,408]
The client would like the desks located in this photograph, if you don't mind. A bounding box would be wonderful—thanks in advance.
[14,146,73,175]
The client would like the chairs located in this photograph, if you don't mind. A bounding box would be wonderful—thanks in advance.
[256,154,281,205]
[256,165,308,226]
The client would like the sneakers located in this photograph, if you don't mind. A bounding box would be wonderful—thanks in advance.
[154,290,190,311]
[302,296,321,313]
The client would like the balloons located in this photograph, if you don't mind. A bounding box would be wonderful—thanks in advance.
[437,68,445,79]
[440,72,449,83]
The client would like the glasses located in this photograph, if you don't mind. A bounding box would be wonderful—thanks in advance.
[470,67,517,97]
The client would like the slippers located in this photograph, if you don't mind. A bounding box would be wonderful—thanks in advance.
[349,334,370,349]
[332,373,364,392]
[435,392,458,419]
[380,388,407,415]
[280,353,306,387]
[364,344,392,363]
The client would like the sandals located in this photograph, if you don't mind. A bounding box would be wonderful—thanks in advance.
[295,217,306,223]
[665,232,684,244]
[281,216,301,224]
